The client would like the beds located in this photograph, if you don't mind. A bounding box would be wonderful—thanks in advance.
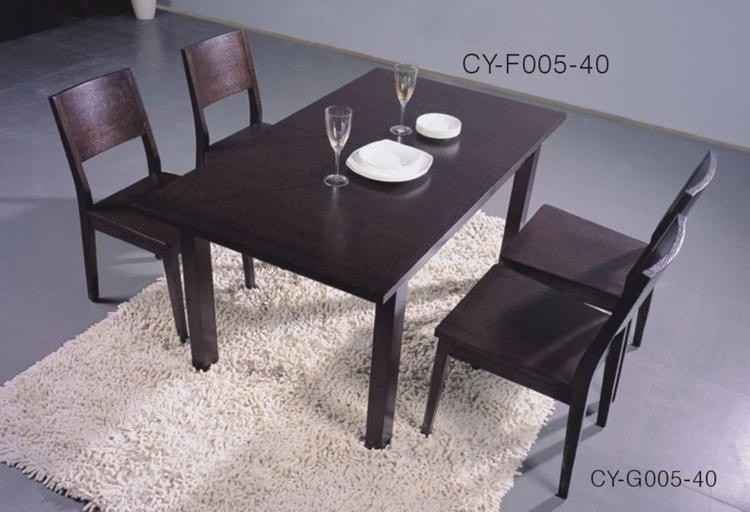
[129,66,569,450]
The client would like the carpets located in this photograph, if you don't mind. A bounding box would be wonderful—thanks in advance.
[1,206,612,512]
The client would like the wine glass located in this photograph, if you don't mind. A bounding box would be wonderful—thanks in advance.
[389,62,418,137]
[322,105,353,187]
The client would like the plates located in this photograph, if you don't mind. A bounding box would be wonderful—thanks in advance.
[344,139,435,184]
[415,113,462,140]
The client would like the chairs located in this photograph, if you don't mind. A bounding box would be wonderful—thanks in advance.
[181,27,273,290]
[48,64,190,345]
[498,148,722,405]
[418,212,690,503]
